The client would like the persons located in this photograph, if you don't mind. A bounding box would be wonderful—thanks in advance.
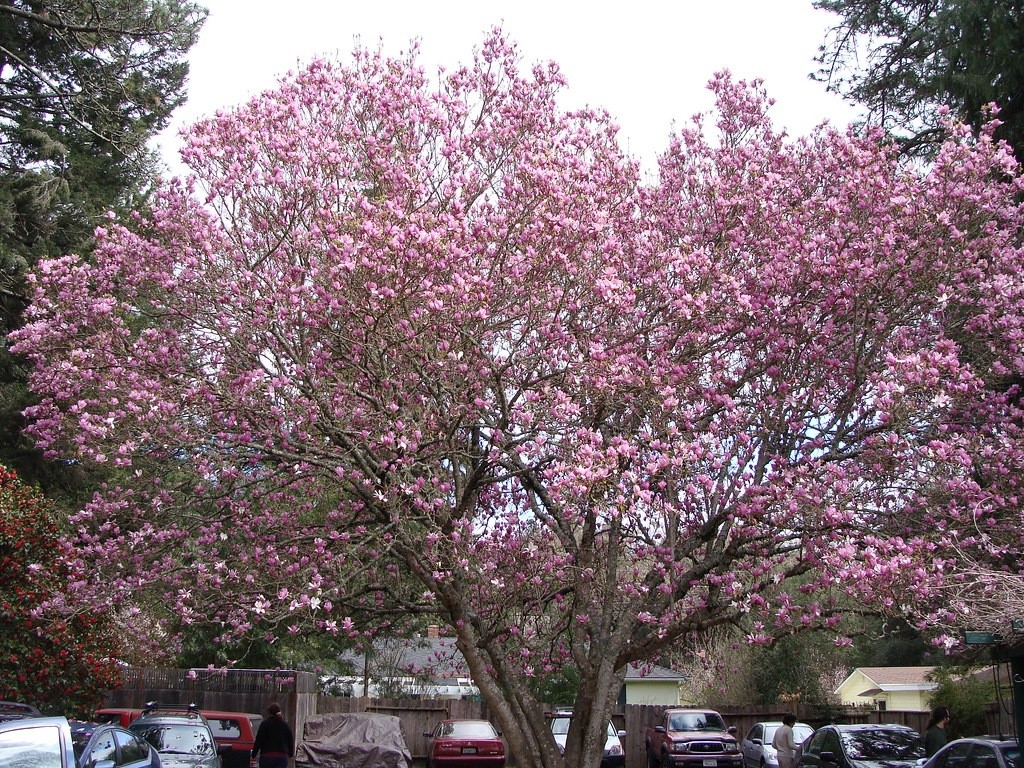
[251,703,294,768]
[772,713,799,768]
[924,705,950,768]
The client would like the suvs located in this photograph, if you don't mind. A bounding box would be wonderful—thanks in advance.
[127,700,233,768]
[546,706,628,768]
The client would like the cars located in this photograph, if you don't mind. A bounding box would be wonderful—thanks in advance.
[793,723,930,768]
[912,734,1024,768]
[742,721,817,768]
[421,719,506,768]
[0,702,165,768]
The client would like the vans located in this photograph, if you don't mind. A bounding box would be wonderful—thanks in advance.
[95,706,263,768]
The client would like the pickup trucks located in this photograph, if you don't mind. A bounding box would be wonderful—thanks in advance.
[644,707,746,768]
